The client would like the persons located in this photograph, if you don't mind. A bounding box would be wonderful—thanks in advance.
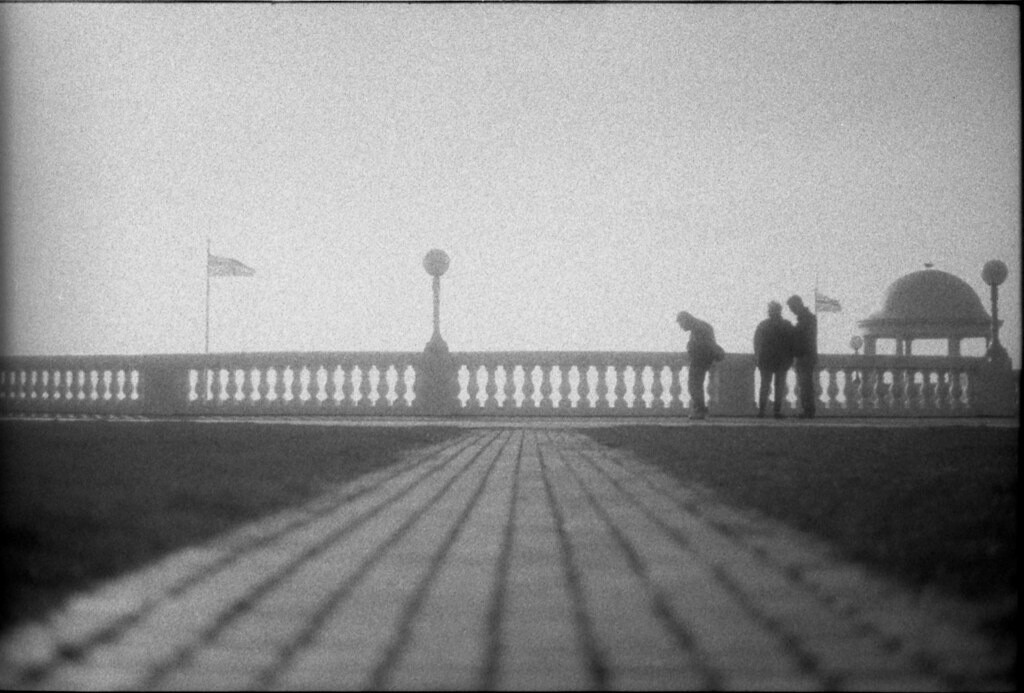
[676,310,717,421]
[753,300,797,419]
[786,294,819,418]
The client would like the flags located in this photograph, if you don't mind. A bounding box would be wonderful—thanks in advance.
[815,293,842,312]
[207,253,256,279]
